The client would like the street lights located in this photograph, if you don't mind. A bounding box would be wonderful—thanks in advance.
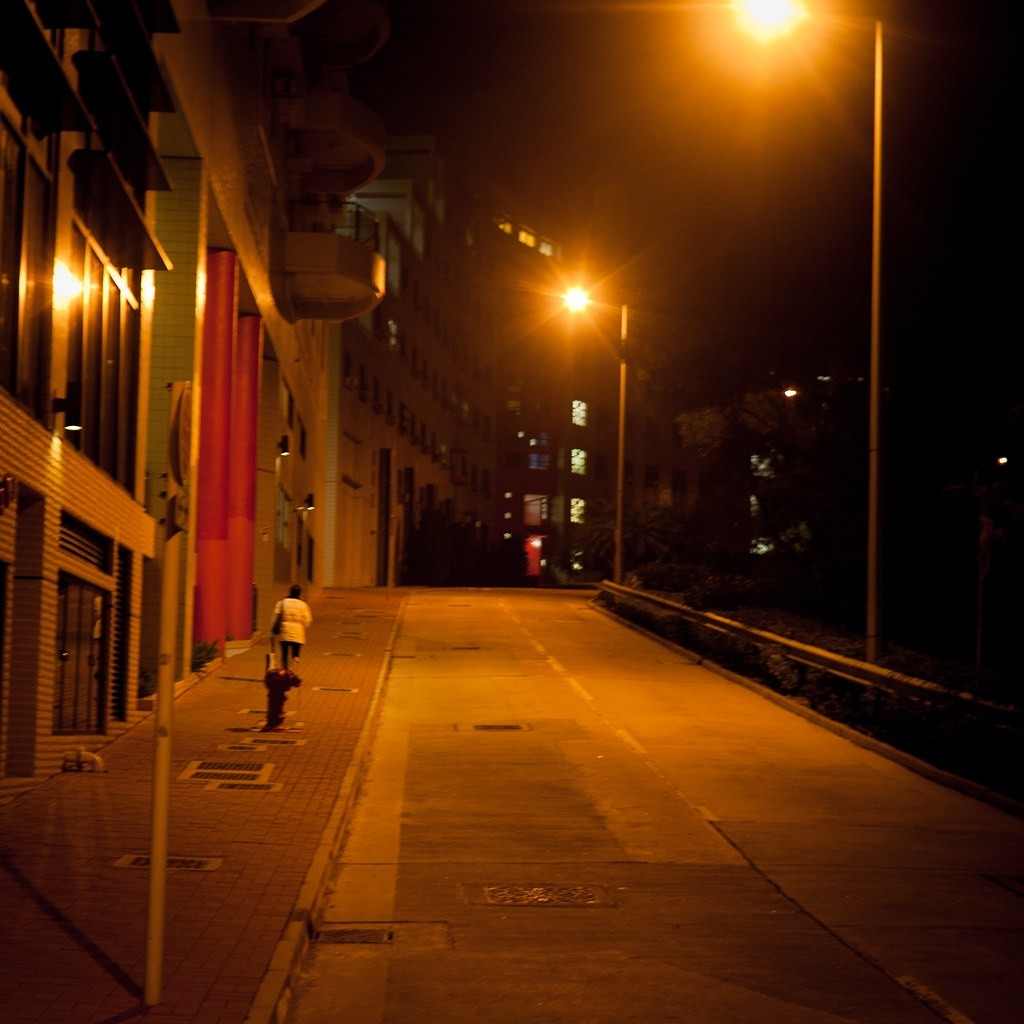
[739,0,882,665]
[562,287,627,585]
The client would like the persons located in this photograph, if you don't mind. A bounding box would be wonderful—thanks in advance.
[272,584,313,671]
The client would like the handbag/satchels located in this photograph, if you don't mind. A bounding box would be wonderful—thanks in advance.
[272,600,283,634]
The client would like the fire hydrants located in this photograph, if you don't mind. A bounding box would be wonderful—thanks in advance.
[265,666,302,731]
